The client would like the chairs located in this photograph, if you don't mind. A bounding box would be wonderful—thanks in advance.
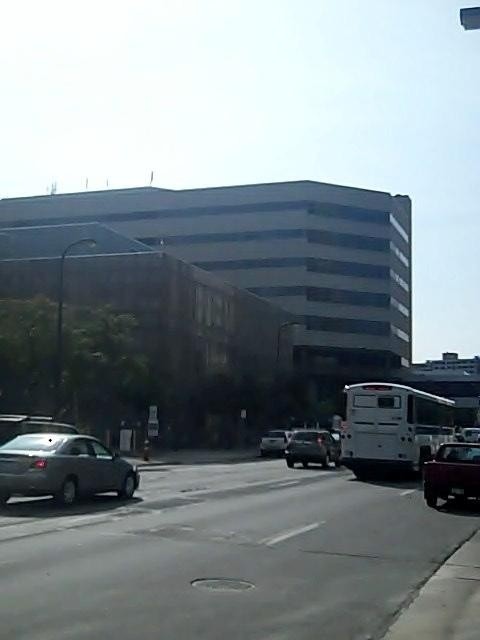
[472,456,480,462]
[447,450,459,459]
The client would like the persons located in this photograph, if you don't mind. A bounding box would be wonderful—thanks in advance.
[455,425,466,443]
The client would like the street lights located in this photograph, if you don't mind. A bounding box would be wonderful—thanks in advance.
[460,6,479,30]
[51,236,97,422]
[271,319,307,432]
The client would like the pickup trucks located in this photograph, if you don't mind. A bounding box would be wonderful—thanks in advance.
[418,440,480,517]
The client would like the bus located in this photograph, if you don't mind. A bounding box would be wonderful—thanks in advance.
[337,378,460,484]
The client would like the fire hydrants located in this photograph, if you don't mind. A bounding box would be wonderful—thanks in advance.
[142,439,153,462]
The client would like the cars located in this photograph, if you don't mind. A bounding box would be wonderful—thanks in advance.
[456,426,479,445]
[0,432,142,510]
[321,429,344,460]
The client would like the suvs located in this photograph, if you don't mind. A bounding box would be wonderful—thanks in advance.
[281,428,341,472]
[0,410,78,446]
[255,429,292,458]
